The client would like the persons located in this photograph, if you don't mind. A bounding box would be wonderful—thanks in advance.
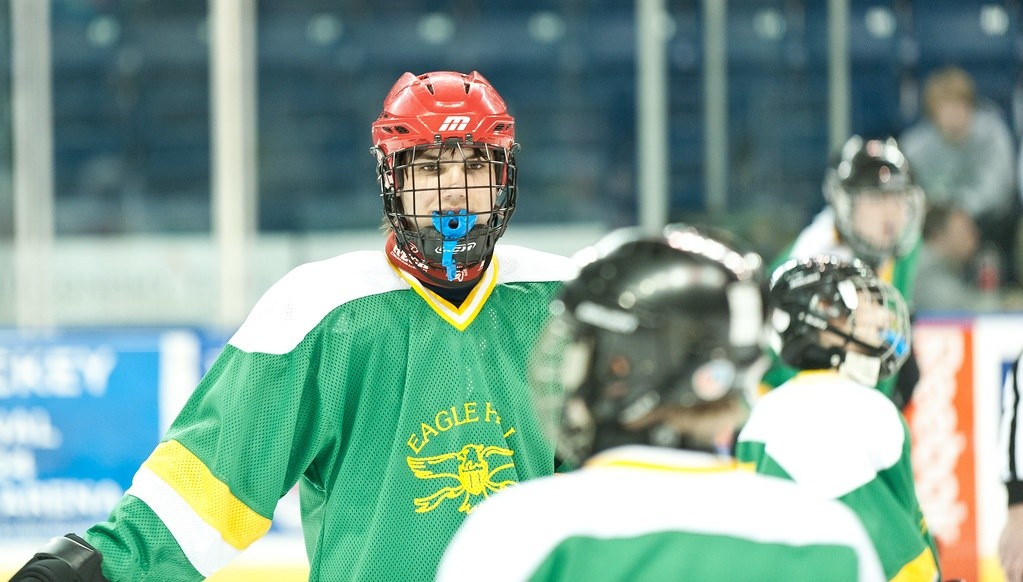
[435,239,887,582]
[756,133,920,429]
[898,68,1020,290]
[8,69,576,582]
[734,253,941,582]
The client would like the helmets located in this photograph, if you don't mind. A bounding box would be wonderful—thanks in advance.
[764,254,913,380]
[533,223,790,464]
[822,135,927,260]
[372,70,521,290]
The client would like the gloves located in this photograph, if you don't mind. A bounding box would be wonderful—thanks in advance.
[8,533,107,582]
